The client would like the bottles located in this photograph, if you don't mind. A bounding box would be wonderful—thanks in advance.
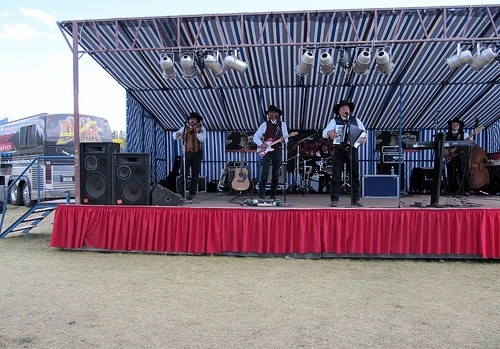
[66,193,70,204]
[83,196,89,204]
[390,166,394,175]
[117,197,122,204]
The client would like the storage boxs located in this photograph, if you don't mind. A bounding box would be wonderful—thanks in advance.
[361,174,400,199]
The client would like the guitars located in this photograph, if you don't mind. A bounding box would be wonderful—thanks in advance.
[231,143,250,191]
[256,131,298,158]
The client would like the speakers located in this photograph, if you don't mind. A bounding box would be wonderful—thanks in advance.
[259,165,286,184]
[80,142,182,206]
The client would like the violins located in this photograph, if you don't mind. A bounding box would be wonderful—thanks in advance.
[182,119,200,135]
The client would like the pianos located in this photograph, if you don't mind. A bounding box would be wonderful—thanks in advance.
[400,139,476,196]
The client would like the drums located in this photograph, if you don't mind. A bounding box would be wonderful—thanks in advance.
[310,156,333,192]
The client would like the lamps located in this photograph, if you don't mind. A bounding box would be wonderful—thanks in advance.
[319,48,335,75]
[445,40,500,71]
[353,47,371,75]
[224,49,249,75]
[375,47,395,75]
[159,52,177,80]
[203,50,227,77]
[295,48,316,77]
[179,52,198,80]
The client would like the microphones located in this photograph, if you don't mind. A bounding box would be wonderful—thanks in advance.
[345,112,349,115]
[277,119,282,123]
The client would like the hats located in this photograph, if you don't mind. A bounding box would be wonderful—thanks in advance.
[333,100,355,115]
[448,118,464,132]
[187,112,202,121]
[265,105,282,117]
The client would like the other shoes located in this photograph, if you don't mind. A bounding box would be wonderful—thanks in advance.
[186,194,196,204]
[465,192,470,195]
[331,200,337,206]
[353,201,363,207]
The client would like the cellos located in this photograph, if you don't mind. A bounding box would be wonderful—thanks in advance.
[456,118,491,195]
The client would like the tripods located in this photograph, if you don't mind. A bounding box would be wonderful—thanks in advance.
[341,163,351,193]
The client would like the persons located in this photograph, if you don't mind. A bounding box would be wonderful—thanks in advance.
[322,99,367,207]
[253,105,289,205]
[166,112,204,201]
[444,117,472,196]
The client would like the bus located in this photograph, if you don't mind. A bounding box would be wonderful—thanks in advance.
[0,113,116,206]
